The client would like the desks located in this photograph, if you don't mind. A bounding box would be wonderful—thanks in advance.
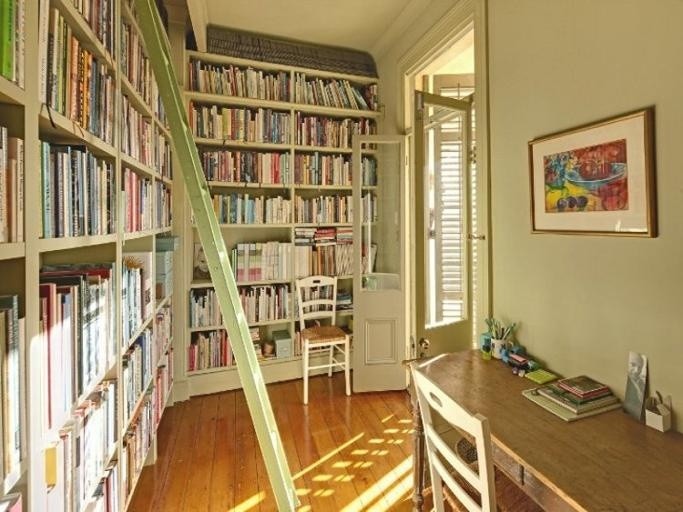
[403,347,683,511]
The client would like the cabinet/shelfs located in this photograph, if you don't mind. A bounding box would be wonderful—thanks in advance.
[180,49,380,401]
[1,2,181,510]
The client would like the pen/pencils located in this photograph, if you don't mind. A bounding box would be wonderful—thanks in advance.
[485,317,517,341]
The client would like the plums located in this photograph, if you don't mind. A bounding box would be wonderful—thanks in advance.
[557,196,587,209]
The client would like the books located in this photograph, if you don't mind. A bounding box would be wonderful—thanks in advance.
[190,320,353,372]
[1,377,119,512]
[189,61,376,149]
[201,150,379,223]
[190,225,377,328]
[118,1,169,129]
[1,1,113,144]
[122,237,177,346]
[6,262,116,476]
[122,93,172,178]
[123,349,174,509]
[520,373,624,423]
[122,168,172,233]
[1,128,114,242]
[122,306,172,427]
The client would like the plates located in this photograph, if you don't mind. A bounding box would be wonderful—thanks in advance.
[564,163,626,190]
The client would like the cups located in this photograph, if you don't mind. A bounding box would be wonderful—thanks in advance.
[478,331,515,361]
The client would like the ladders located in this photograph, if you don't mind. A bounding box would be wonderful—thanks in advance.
[134,0,302,512]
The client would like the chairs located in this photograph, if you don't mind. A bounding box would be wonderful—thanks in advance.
[294,276,353,405]
[408,361,498,512]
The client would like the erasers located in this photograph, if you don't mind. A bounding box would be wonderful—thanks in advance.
[512,367,525,377]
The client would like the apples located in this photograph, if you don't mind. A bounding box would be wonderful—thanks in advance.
[575,143,628,211]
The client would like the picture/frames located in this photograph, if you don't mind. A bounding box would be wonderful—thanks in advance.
[527,104,657,238]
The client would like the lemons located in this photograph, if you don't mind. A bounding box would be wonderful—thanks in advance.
[545,188,561,209]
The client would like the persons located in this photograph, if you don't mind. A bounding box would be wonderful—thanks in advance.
[626,351,645,414]
